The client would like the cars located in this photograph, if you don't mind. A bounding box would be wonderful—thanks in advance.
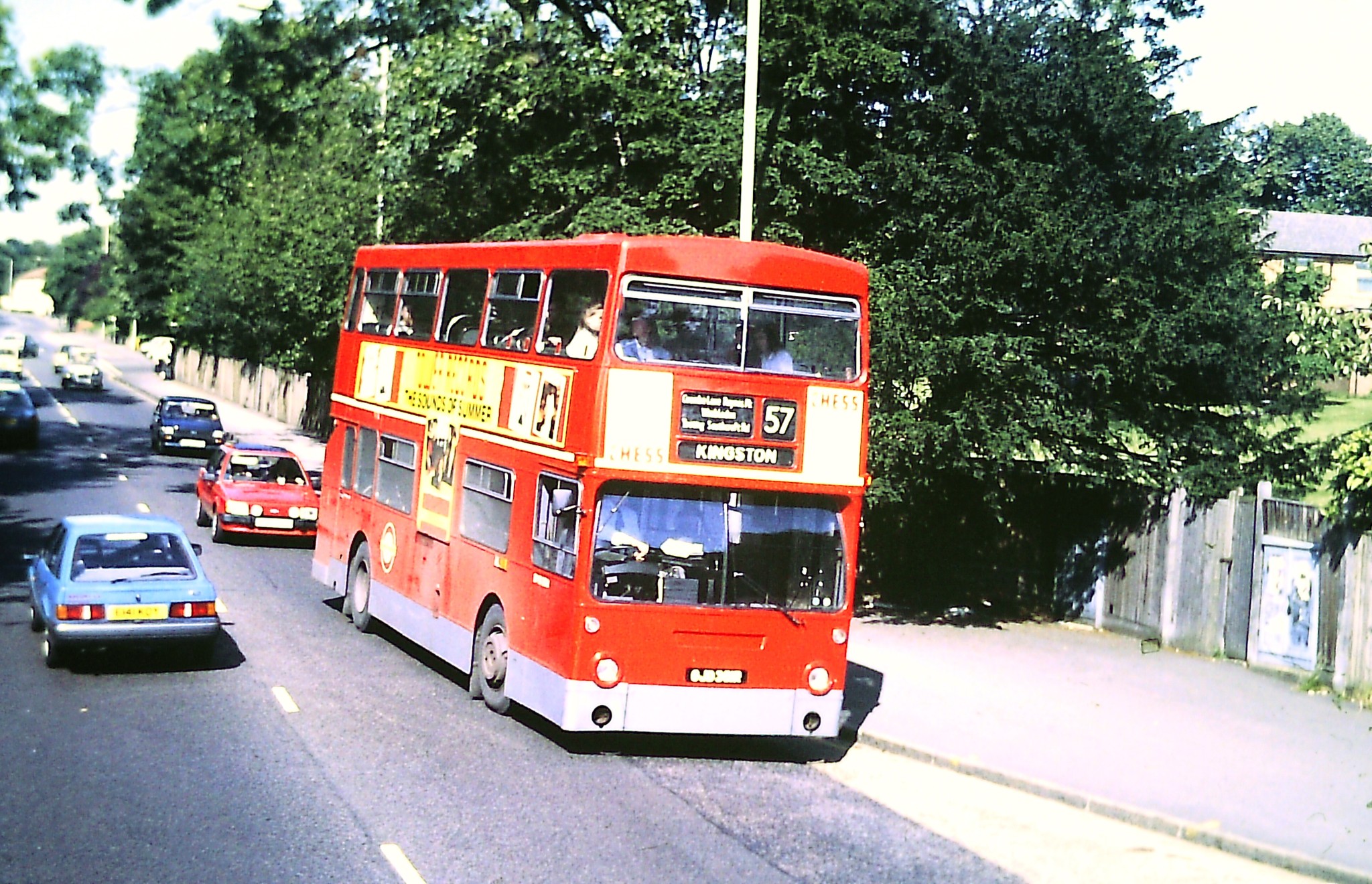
[150,396,226,458]
[17,514,222,667]
[52,343,106,392]
[0,330,42,434]
[194,441,320,550]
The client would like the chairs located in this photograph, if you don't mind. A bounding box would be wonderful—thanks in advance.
[363,314,577,358]
[169,405,182,416]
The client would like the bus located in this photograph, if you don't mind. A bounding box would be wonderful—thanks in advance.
[309,232,873,736]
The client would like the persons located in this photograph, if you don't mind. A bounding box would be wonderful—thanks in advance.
[391,292,793,374]
[599,494,642,543]
